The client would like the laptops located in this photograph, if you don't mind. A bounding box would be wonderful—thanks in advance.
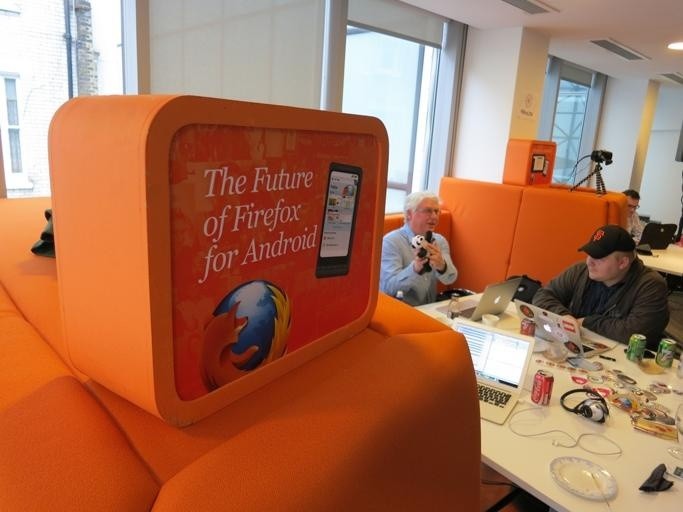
[636,222,677,249]
[512,298,611,358]
[450,317,535,424]
[436,278,526,322]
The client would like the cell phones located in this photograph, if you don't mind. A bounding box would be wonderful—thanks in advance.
[315,162,363,277]
[543,160,549,176]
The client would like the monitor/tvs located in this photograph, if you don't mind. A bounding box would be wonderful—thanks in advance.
[532,154,545,172]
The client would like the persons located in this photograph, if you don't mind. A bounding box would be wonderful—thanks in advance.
[380,188,459,308]
[621,189,643,246]
[531,223,671,353]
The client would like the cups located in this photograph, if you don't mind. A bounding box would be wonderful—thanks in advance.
[481,313,500,329]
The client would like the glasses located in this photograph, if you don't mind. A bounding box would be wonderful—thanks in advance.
[628,205,640,209]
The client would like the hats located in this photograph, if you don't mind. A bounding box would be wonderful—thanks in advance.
[576,224,636,259]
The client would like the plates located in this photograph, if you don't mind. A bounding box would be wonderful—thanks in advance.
[548,456,618,502]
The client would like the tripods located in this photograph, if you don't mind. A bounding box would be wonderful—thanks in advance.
[569,165,606,195]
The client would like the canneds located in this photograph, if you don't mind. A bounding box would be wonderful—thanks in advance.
[655,338,677,368]
[521,318,536,337]
[531,370,554,406]
[626,334,646,362]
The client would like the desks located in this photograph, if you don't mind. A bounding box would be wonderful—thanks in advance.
[411,241,683,512]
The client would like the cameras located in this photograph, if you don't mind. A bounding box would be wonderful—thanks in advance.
[591,150,613,165]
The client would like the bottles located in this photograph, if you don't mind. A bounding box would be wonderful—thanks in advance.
[446,292,460,321]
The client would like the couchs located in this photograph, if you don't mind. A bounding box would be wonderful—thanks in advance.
[1,180,641,510]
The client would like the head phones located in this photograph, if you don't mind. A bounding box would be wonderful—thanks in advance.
[560,388,609,424]
